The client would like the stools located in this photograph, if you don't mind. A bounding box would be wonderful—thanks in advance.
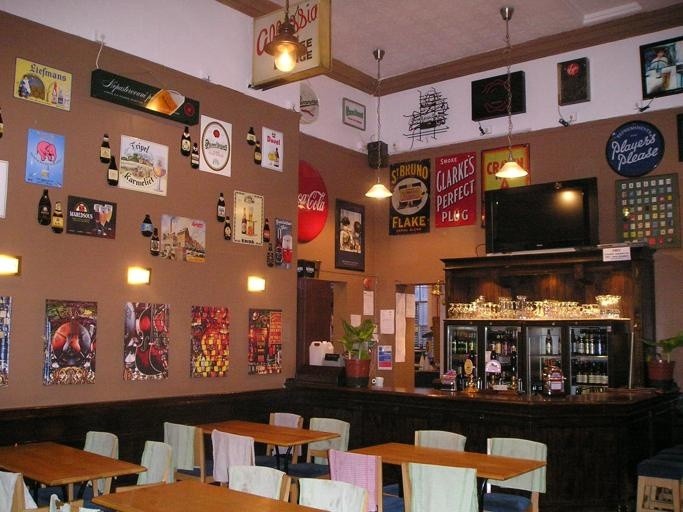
[635,437,683,512]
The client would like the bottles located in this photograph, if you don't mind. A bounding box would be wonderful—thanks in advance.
[50,201,64,235]
[241,207,254,236]
[100,134,119,186]
[542,358,566,397]
[262,217,282,268]
[36,187,52,226]
[48,80,63,106]
[273,148,279,167]
[139,214,161,258]
[245,127,262,165]
[450,329,517,389]
[518,378,522,391]
[419,353,425,365]
[215,191,232,241]
[570,327,607,386]
[661,66,676,90]
[178,125,200,170]
[545,329,553,354]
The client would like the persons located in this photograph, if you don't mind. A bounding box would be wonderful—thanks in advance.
[338,216,353,249]
[648,48,667,70]
[352,221,360,250]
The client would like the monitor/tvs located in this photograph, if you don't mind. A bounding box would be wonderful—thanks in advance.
[485,176,599,257]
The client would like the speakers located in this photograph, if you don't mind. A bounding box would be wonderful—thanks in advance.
[368,141,387,168]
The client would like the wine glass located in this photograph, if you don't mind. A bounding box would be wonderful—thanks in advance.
[92,204,113,237]
[447,293,622,320]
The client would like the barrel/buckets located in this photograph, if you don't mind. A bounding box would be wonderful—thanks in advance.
[309,341,334,367]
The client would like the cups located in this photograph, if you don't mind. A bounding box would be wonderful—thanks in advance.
[143,90,186,115]
[281,234,292,264]
[372,376,385,387]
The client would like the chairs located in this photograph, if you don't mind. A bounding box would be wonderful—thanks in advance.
[0,405,550,512]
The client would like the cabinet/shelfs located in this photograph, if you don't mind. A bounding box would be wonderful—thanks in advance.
[442,319,631,395]
[440,242,655,365]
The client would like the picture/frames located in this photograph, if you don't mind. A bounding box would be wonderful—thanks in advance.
[556,55,590,106]
[334,198,367,272]
[638,34,682,100]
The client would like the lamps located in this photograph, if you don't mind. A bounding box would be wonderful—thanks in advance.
[493,18,525,183]
[264,0,308,72]
[362,49,392,202]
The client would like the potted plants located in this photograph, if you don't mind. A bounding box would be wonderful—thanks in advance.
[638,328,682,388]
[332,315,377,388]
